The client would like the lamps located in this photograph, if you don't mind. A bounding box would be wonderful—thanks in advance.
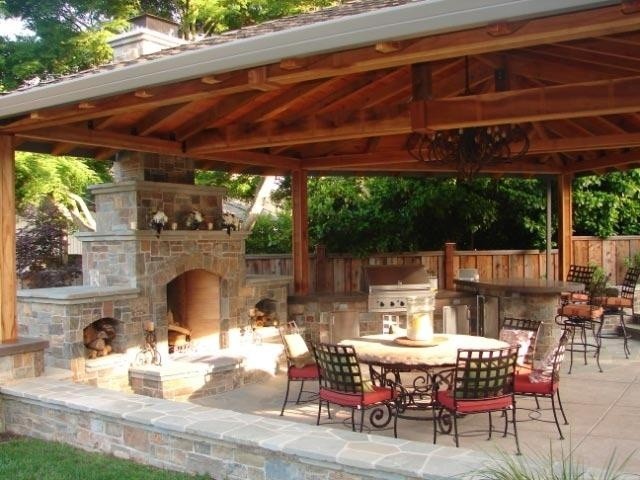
[405,53,532,188]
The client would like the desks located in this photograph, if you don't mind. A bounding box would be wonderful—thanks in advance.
[338,334,511,436]
[453,272,589,359]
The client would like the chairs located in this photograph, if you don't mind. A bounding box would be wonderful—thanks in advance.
[561,262,640,375]
[433,346,522,457]
[379,313,544,414]
[310,341,400,440]
[502,322,569,441]
[280,320,330,418]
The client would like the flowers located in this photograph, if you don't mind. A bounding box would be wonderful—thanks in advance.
[150,207,237,240]
